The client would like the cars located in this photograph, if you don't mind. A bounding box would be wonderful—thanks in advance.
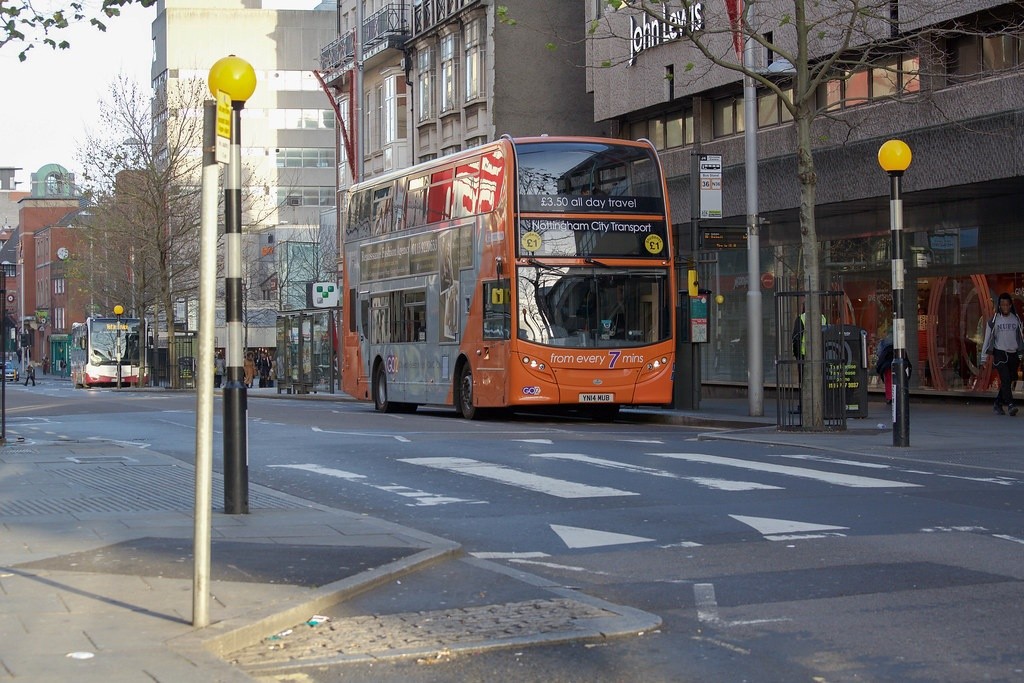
[0,360,20,381]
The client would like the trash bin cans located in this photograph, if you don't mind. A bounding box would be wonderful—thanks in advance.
[821,323,870,419]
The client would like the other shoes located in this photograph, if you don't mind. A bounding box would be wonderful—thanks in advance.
[789,409,800,413]
[991,402,1005,415]
[1008,403,1019,416]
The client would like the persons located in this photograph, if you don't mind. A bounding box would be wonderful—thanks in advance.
[789,301,827,414]
[877,333,913,404]
[979,293,1024,416]
[215,352,225,388]
[17,348,22,363]
[244,351,277,388]
[60,357,67,378]
[23,363,35,386]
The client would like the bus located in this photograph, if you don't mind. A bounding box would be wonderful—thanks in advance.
[340,134,700,421]
[72,315,148,389]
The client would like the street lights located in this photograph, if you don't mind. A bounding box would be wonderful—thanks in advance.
[207,56,256,516]
[877,139,911,446]
[742,60,798,417]
[114,305,124,389]
